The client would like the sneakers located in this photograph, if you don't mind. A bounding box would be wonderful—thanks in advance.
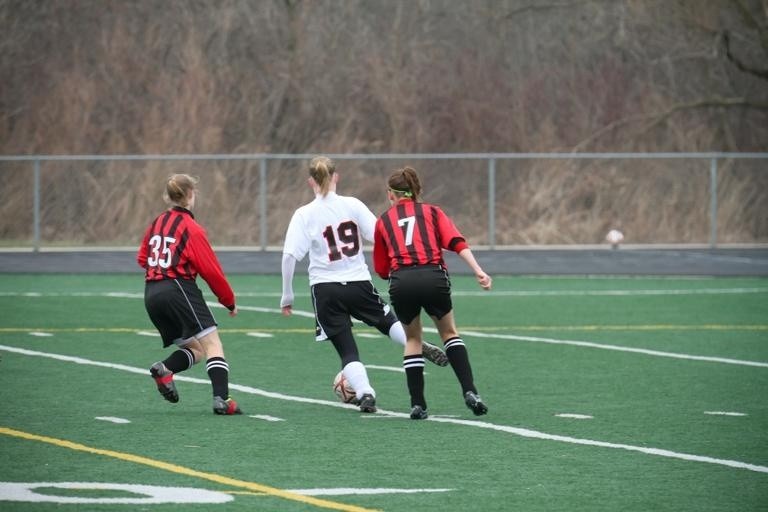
[465,391,488,416]
[409,405,428,419]
[213,395,243,414]
[422,340,449,367]
[358,393,377,413]
[150,361,179,403]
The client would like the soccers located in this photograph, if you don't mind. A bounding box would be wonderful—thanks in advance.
[335,370,358,404]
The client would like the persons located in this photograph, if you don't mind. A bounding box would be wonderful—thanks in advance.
[373,166,492,419]
[136,172,244,414]
[280,155,449,413]
